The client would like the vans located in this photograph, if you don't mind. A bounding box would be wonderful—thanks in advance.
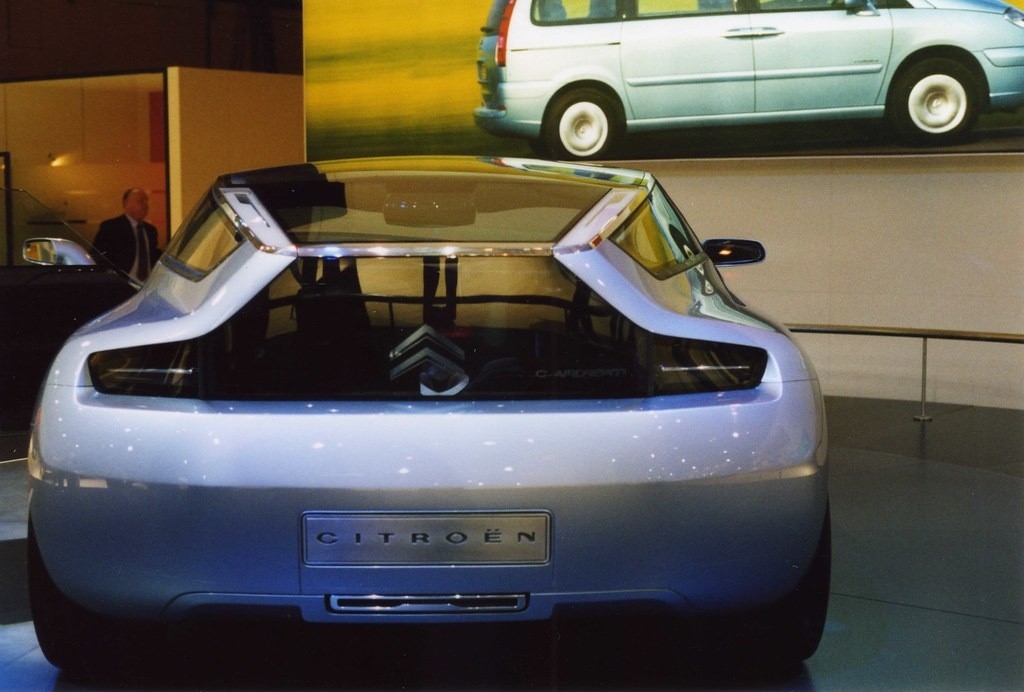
[474,0,1024,160]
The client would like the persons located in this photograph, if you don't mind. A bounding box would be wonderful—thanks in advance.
[92,188,163,282]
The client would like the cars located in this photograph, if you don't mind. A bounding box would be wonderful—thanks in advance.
[20,155,833,671]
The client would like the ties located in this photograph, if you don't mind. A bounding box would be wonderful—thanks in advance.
[135,224,149,282]
